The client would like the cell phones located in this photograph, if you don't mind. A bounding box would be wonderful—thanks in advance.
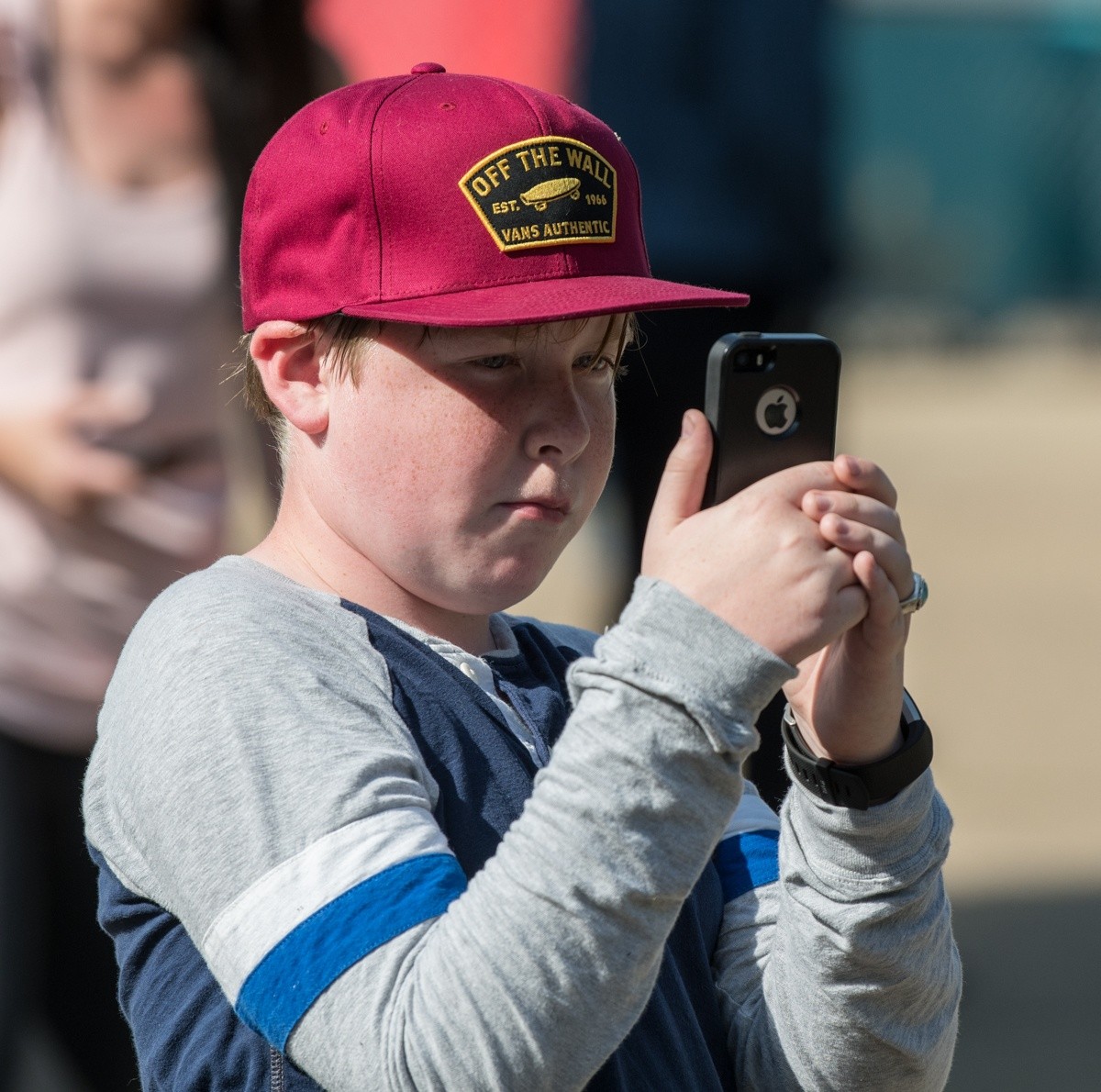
[698,332,841,509]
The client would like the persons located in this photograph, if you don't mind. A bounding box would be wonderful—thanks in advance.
[81,61,965,1090]
[0,1,361,1092]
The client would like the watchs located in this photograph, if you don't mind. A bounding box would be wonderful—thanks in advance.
[780,686,934,811]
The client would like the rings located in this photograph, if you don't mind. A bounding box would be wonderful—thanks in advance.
[899,571,929,615]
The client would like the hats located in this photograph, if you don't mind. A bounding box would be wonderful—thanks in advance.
[238,62,750,334]
